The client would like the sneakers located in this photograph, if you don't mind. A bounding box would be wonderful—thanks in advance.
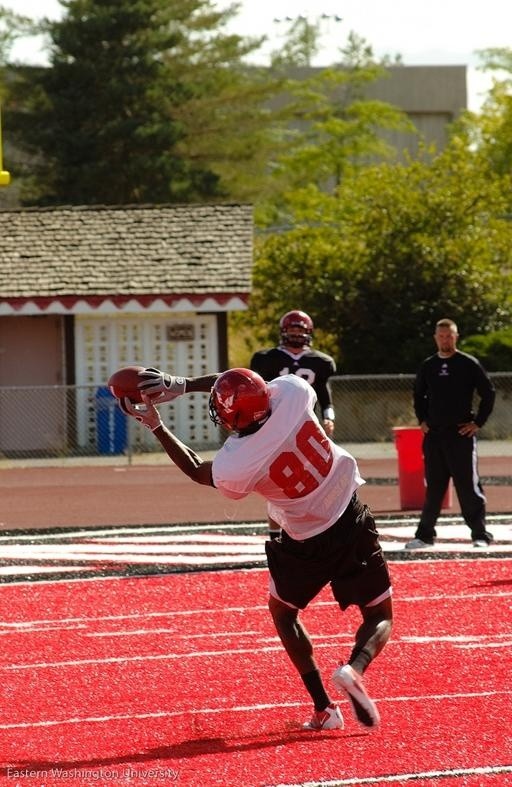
[404,538,432,549]
[303,702,344,731]
[331,666,381,725]
[473,540,489,547]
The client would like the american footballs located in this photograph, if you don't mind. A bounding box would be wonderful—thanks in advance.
[107,366,162,404]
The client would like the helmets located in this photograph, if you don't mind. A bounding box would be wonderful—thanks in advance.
[279,310,314,348]
[206,367,269,433]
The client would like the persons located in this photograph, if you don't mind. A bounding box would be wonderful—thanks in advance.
[115,368,394,731]
[405,317,498,551]
[250,307,339,540]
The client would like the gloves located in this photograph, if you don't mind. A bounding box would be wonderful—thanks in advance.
[138,368,186,405]
[119,395,163,433]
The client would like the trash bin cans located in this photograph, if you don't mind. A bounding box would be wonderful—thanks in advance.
[95,386,128,456]
[392,427,452,512]
